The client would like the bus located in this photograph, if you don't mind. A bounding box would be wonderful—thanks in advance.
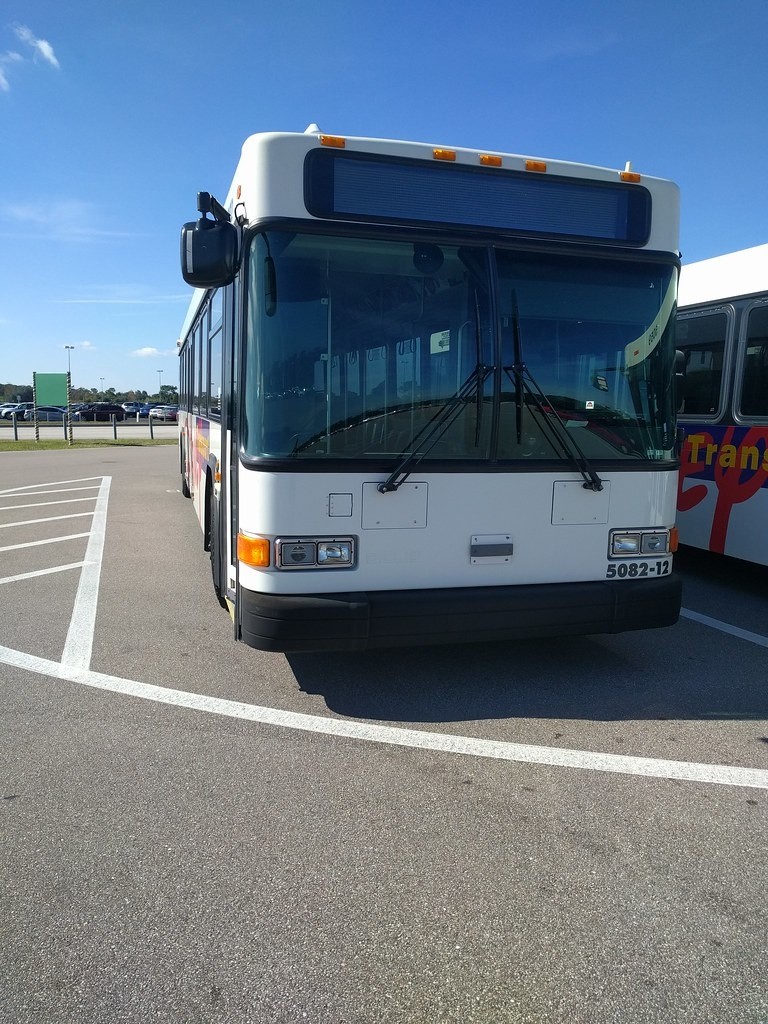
[675,244,768,576]
[177,123,682,652]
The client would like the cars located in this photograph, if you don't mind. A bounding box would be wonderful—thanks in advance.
[0,400,177,422]
